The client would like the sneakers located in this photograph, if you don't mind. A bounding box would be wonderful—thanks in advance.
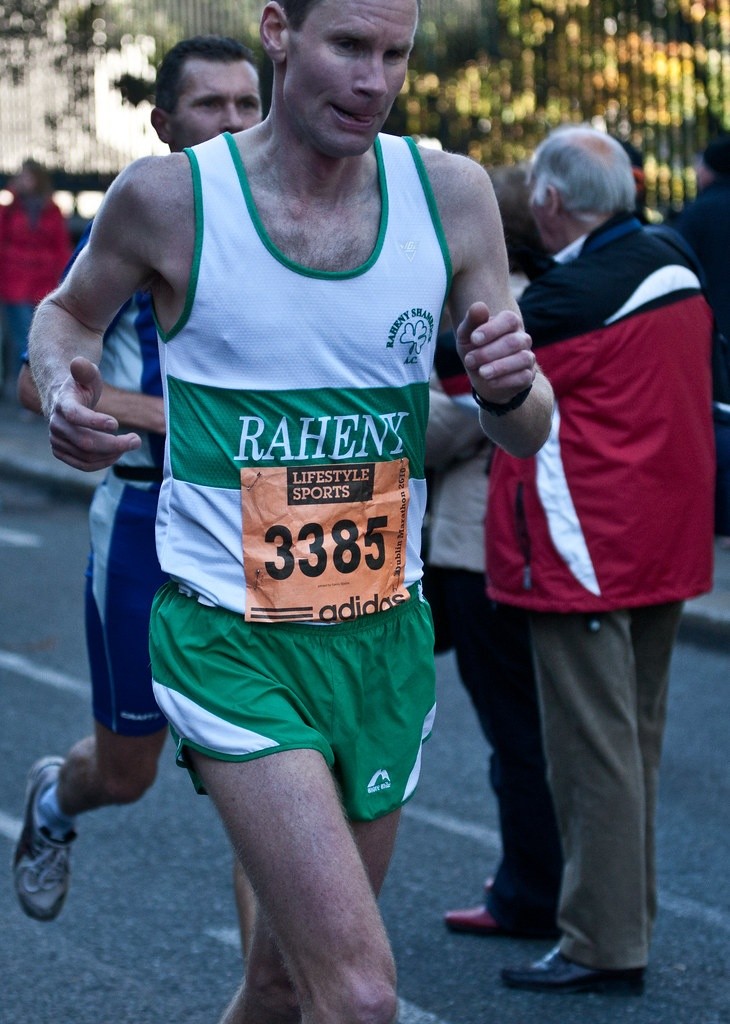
[12,755,78,922]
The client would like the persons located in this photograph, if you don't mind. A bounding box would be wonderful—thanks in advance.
[434,127,713,993]
[25,0,554,1024]
[421,164,564,938]
[1,158,72,424]
[14,37,263,922]
[664,132,730,538]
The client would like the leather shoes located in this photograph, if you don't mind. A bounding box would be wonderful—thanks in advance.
[501,944,645,997]
[445,878,555,939]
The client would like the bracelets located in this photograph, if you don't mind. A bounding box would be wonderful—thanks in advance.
[471,386,531,416]
